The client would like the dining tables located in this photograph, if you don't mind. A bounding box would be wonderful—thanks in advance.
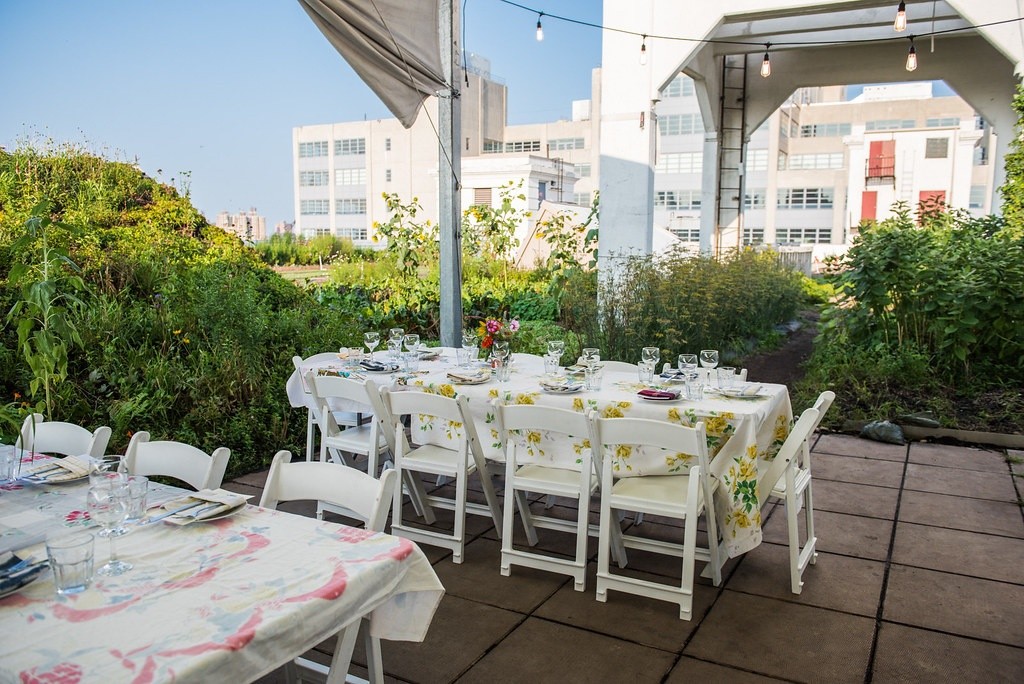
[0,441,445,684]
[286,343,796,576]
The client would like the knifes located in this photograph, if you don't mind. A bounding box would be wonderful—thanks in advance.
[139,501,206,525]
[0,465,60,486]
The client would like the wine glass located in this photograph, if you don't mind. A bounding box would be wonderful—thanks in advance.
[547,340,600,382]
[363,327,419,363]
[86,480,134,577]
[456,335,510,383]
[641,346,719,398]
[87,454,130,538]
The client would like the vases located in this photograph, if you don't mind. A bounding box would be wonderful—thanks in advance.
[491,342,510,374]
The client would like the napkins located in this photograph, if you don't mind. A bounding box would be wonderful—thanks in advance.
[0,533,50,596]
[446,372,490,383]
[565,364,604,371]
[21,454,111,487]
[538,380,586,391]
[415,349,444,358]
[156,488,257,527]
[638,390,681,400]
[660,370,699,380]
[360,361,399,371]
[724,384,772,396]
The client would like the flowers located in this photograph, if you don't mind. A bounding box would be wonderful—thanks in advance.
[474,316,520,361]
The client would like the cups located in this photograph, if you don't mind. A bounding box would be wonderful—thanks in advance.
[0,443,16,488]
[717,366,736,391]
[543,353,560,376]
[404,351,418,372]
[684,373,705,401]
[116,476,149,527]
[348,346,364,366]
[585,367,602,393]
[45,532,95,596]
[638,361,652,384]
[386,339,402,361]
[495,358,510,383]
[457,348,470,368]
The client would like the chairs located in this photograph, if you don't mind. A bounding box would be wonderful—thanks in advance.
[14,369,837,684]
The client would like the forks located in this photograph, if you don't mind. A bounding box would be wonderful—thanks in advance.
[169,504,222,520]
[22,470,72,481]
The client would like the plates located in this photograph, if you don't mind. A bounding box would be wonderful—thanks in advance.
[722,393,759,398]
[366,368,399,375]
[22,476,89,485]
[449,376,491,385]
[638,393,687,402]
[0,574,40,599]
[160,499,247,523]
[542,387,584,394]
